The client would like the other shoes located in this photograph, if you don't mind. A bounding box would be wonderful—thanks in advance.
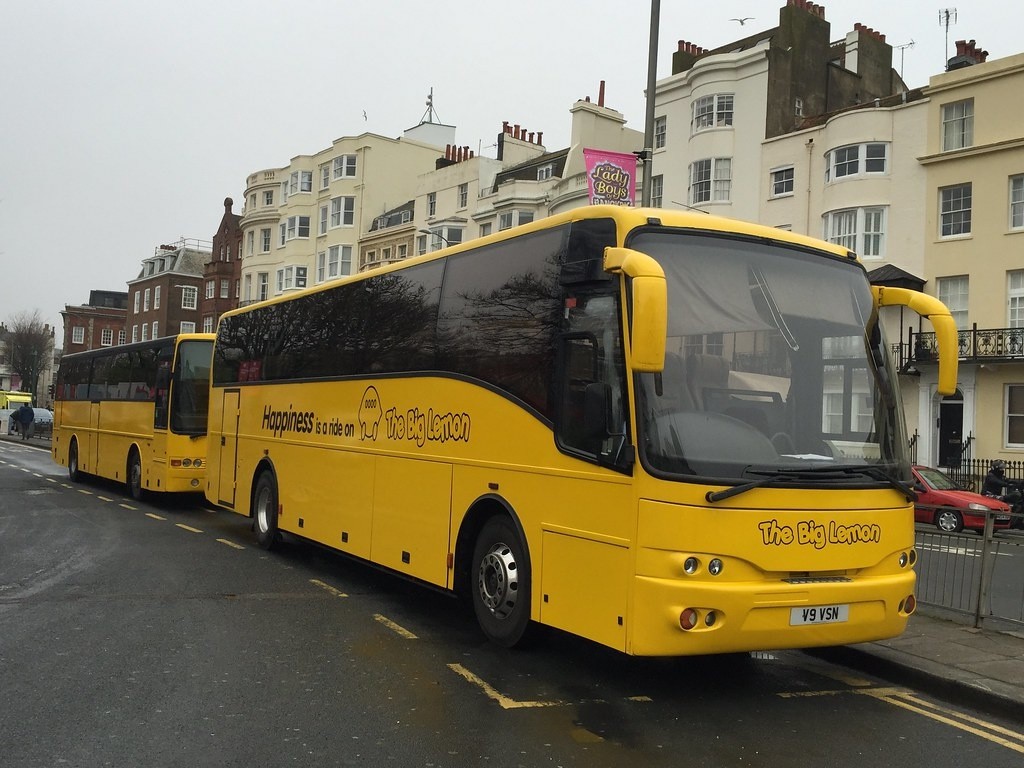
[22,433,29,440]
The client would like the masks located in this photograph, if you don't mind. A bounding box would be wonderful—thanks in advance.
[999,470,1004,476]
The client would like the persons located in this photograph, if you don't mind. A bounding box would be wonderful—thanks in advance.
[981,460,1019,501]
[8,406,24,435]
[19,402,35,440]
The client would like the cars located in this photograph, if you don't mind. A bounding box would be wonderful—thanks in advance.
[14,408,53,432]
[909,465,1010,534]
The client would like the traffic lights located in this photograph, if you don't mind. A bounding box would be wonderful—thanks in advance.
[48,385,54,394]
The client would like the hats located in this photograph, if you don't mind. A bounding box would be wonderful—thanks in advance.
[992,461,1006,469]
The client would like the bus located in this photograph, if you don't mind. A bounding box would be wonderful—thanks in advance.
[51,332,216,500]
[204,204,958,658]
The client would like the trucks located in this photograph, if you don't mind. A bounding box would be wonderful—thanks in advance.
[0,391,33,410]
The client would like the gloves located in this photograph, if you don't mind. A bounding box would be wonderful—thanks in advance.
[1009,484,1017,488]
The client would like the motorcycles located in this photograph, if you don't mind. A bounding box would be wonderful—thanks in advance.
[1003,483,1024,530]
